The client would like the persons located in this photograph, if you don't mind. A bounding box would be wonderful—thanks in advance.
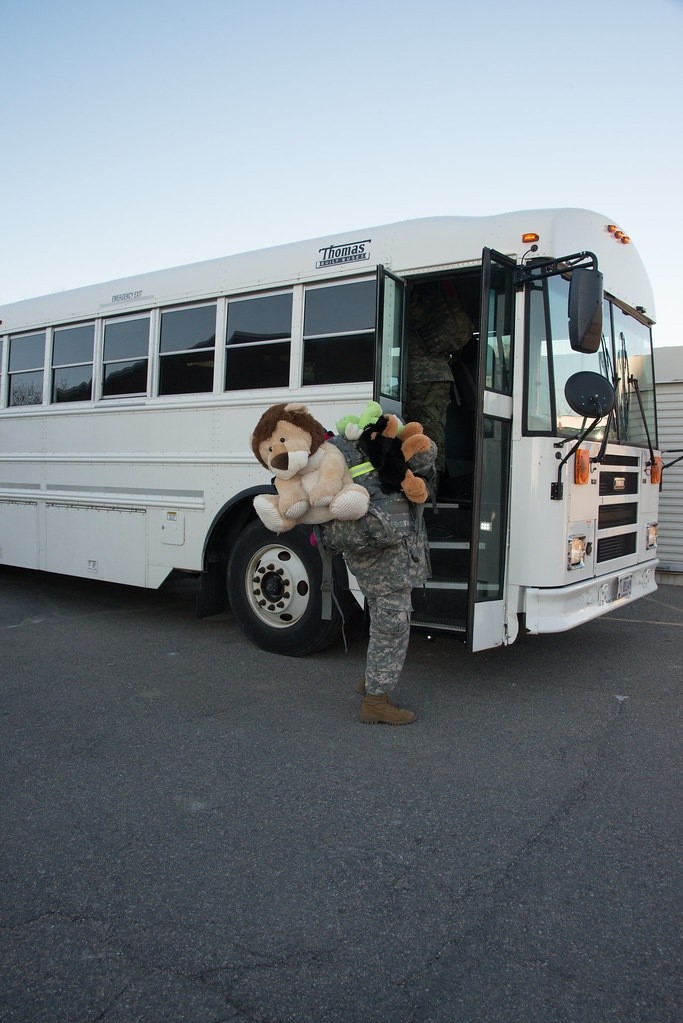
[278,350,318,385]
[403,288,455,501]
[341,431,433,724]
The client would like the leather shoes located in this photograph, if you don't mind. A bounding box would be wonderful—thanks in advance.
[353,676,365,696]
[359,693,417,725]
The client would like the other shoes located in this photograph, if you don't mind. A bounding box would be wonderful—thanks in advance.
[426,520,457,539]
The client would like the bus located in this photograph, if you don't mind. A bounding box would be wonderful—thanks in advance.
[0,207,683,655]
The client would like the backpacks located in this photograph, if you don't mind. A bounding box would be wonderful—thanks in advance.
[408,281,476,358]
[312,434,416,556]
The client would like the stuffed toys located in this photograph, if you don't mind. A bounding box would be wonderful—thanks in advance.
[252,403,372,534]
[336,401,437,504]
